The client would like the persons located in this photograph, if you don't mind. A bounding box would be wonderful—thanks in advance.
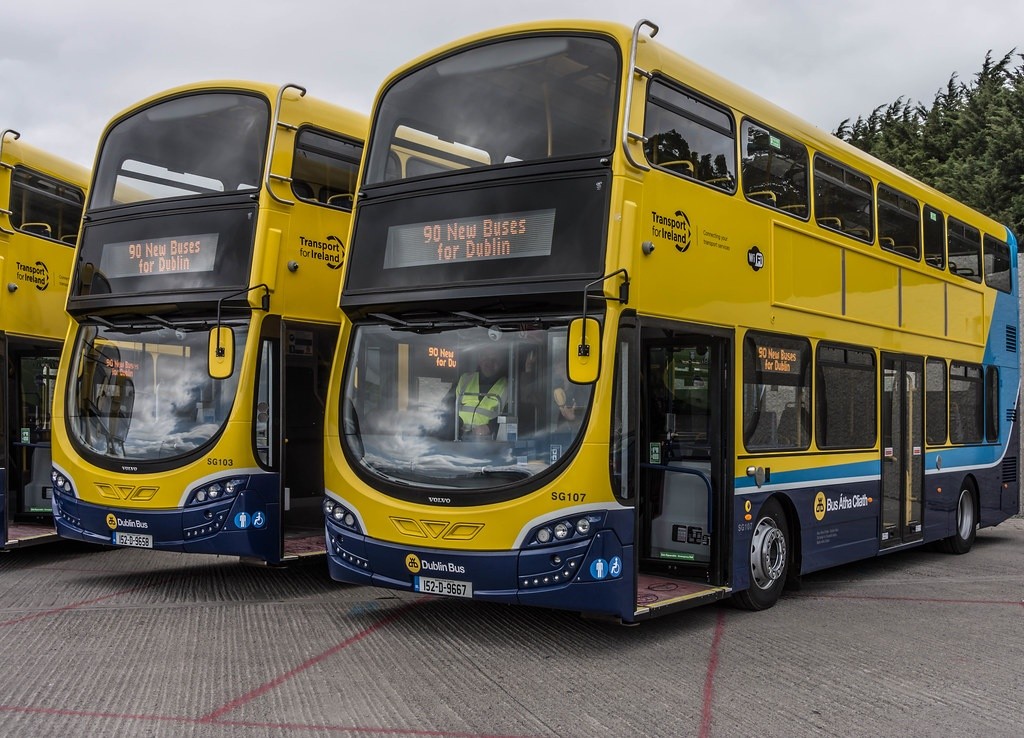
[520,350,586,441]
[435,343,513,442]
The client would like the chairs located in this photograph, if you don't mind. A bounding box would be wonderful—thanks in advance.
[20,223,77,245]
[929,398,963,441]
[327,192,353,209]
[654,158,975,277]
[744,392,880,445]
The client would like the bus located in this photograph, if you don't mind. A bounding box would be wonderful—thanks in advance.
[48,81,802,564]
[1,131,165,549]
[322,17,1024,626]
